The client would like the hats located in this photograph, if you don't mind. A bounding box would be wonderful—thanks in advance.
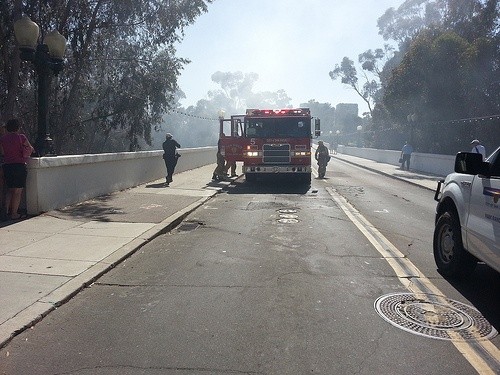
[470,139,480,144]
[165,133,173,138]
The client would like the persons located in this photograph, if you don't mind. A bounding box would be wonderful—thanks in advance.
[315,140,330,177]
[212,133,226,179]
[328,139,339,155]
[0,119,34,221]
[400,140,414,171]
[470,139,486,161]
[163,133,181,186]
[224,132,239,176]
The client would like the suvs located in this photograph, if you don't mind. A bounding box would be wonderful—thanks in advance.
[433,145,500,275]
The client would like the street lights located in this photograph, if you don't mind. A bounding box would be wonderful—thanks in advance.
[407,113,417,152]
[14,15,67,156]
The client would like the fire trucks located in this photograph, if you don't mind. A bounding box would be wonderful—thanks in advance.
[219,108,320,186]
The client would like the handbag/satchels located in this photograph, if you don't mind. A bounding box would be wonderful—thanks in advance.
[19,135,31,159]
[399,158,405,167]
